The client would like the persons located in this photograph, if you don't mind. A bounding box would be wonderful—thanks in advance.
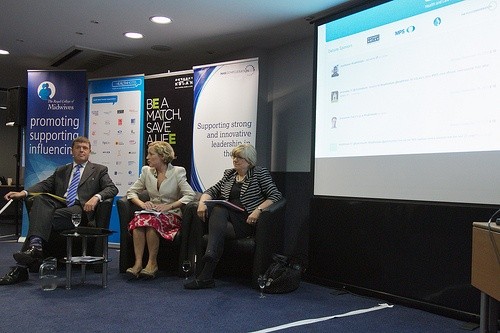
[0,136,119,284]
[126,141,196,279]
[184,143,282,290]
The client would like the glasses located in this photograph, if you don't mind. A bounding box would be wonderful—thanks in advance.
[230,155,245,160]
[145,152,160,157]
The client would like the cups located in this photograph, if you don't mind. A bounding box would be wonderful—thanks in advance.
[7,178,12,186]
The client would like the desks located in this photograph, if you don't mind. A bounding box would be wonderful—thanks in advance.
[59,231,113,290]
[0,185,24,236]
[471,222,500,333]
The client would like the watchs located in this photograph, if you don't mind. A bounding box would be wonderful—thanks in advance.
[257,206,263,212]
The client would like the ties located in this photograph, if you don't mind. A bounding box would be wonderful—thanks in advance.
[66,164,83,207]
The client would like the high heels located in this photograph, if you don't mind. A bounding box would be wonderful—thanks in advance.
[140,265,158,278]
[126,267,143,278]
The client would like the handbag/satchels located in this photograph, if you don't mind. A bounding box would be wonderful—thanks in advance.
[251,257,306,294]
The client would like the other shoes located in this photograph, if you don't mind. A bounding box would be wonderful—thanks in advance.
[184,278,215,289]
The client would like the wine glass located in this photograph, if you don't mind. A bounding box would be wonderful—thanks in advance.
[257,278,266,298]
[182,261,191,281]
[72,213,81,236]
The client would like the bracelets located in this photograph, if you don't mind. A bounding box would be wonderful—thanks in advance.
[170,204,174,209]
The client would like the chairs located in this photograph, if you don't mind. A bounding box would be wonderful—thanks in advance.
[201,171,316,289]
[117,191,199,279]
[25,196,113,273]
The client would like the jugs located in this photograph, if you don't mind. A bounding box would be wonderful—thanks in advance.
[39,257,58,291]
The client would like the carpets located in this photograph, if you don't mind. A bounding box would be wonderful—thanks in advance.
[0,222,478,333]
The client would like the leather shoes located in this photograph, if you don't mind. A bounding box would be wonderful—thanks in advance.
[0,266,29,286]
[13,246,43,265]
